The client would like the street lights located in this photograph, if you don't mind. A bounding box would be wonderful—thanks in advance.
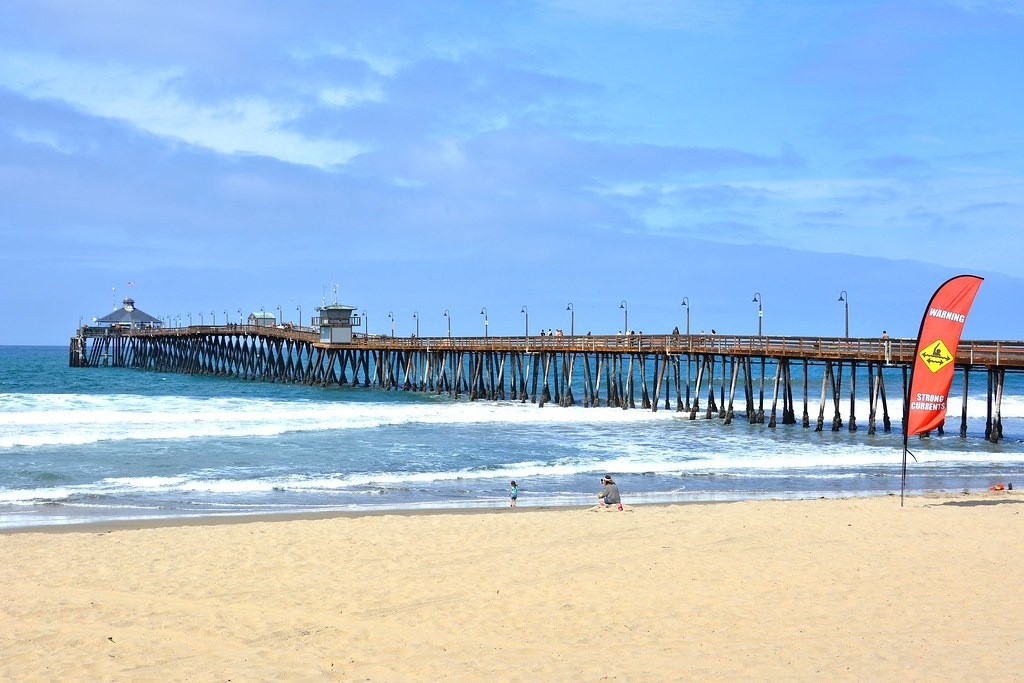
[158,313,181,328]
[620,300,627,335]
[566,303,573,336]
[362,310,367,337]
[413,311,418,338]
[199,312,203,326]
[480,308,488,345]
[838,291,850,352]
[388,311,393,337]
[521,305,528,346]
[443,310,450,337]
[224,310,228,324]
[296,304,301,330]
[188,313,193,326]
[277,305,282,326]
[752,293,762,348]
[681,297,689,347]
[238,308,242,329]
[211,311,215,326]
[260,307,265,327]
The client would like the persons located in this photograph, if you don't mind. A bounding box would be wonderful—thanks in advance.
[541,329,545,336]
[626,329,630,347]
[638,331,643,347]
[353,334,356,342]
[669,326,679,347]
[596,474,621,508]
[556,329,565,343]
[271,322,276,327]
[548,329,552,341]
[230,322,233,331]
[411,333,415,345]
[587,332,591,335]
[882,330,889,337]
[617,331,622,343]
[509,481,519,507]
[709,329,716,346]
[226,322,229,331]
[363,335,368,345]
[699,329,705,344]
[289,321,293,333]
[234,322,237,331]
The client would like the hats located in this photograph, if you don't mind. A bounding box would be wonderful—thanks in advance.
[603,477,614,484]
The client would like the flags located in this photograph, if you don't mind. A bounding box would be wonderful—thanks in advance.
[128,281,135,287]
[907,274,985,435]
